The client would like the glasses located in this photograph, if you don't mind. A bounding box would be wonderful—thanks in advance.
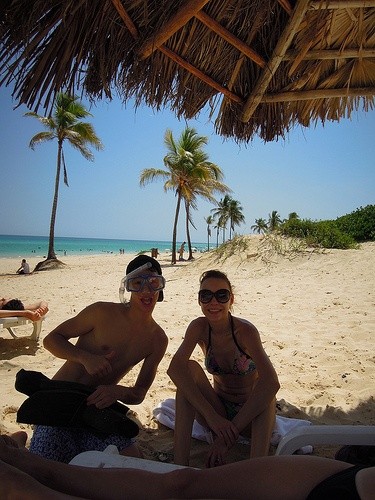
[125,275,165,292]
[198,289,230,304]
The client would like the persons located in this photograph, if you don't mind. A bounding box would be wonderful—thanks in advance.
[29,254,169,462]
[16,240,186,274]
[0,431,375,500]
[166,270,280,467]
[0,297,49,322]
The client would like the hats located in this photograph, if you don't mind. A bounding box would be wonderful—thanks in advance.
[126,255,164,302]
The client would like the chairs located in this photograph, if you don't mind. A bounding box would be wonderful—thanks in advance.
[69,426,375,473]
[0,315,46,341]
